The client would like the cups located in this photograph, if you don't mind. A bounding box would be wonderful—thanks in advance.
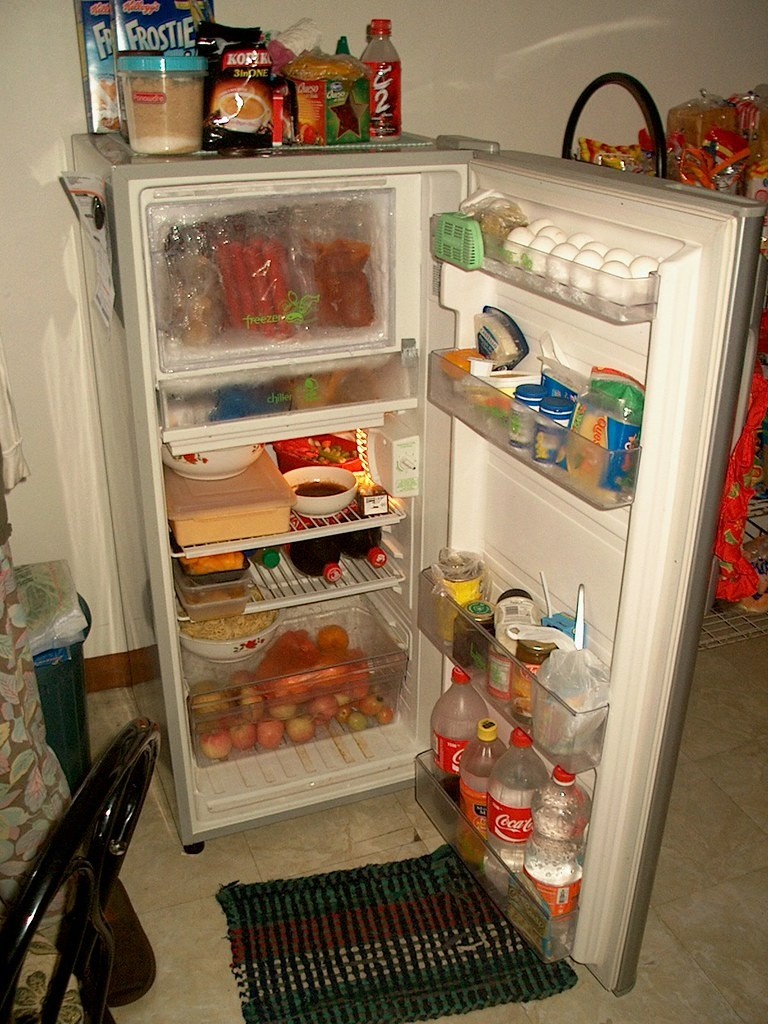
[116,55,209,153]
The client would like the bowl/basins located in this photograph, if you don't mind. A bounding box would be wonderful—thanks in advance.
[162,443,265,481]
[282,465,358,519]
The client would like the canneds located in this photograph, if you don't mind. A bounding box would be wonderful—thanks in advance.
[436,569,558,726]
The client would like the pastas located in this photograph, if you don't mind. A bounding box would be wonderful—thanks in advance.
[176,586,279,641]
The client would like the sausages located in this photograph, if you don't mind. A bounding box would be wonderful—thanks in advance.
[217,236,296,336]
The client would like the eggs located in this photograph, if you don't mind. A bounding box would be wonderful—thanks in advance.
[504,219,661,306]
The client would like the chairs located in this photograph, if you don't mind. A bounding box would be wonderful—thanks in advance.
[0,715,162,1024]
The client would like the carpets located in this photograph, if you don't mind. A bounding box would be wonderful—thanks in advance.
[214,839,579,1024]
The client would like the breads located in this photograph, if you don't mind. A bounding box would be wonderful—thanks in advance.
[567,411,618,504]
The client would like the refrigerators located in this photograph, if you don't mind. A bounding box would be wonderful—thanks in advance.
[68,130,767,1000]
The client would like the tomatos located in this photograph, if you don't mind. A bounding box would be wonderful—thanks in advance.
[335,682,393,731]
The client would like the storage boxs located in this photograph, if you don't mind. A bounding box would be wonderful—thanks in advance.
[161,447,297,547]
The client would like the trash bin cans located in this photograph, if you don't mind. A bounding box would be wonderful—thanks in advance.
[31,592,92,803]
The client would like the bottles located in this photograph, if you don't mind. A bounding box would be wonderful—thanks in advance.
[485,727,550,888]
[456,718,508,866]
[256,546,281,568]
[334,526,388,568]
[430,666,490,826]
[522,765,593,937]
[284,537,342,583]
[358,18,402,142]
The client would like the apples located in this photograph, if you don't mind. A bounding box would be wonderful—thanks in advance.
[200,624,349,758]
